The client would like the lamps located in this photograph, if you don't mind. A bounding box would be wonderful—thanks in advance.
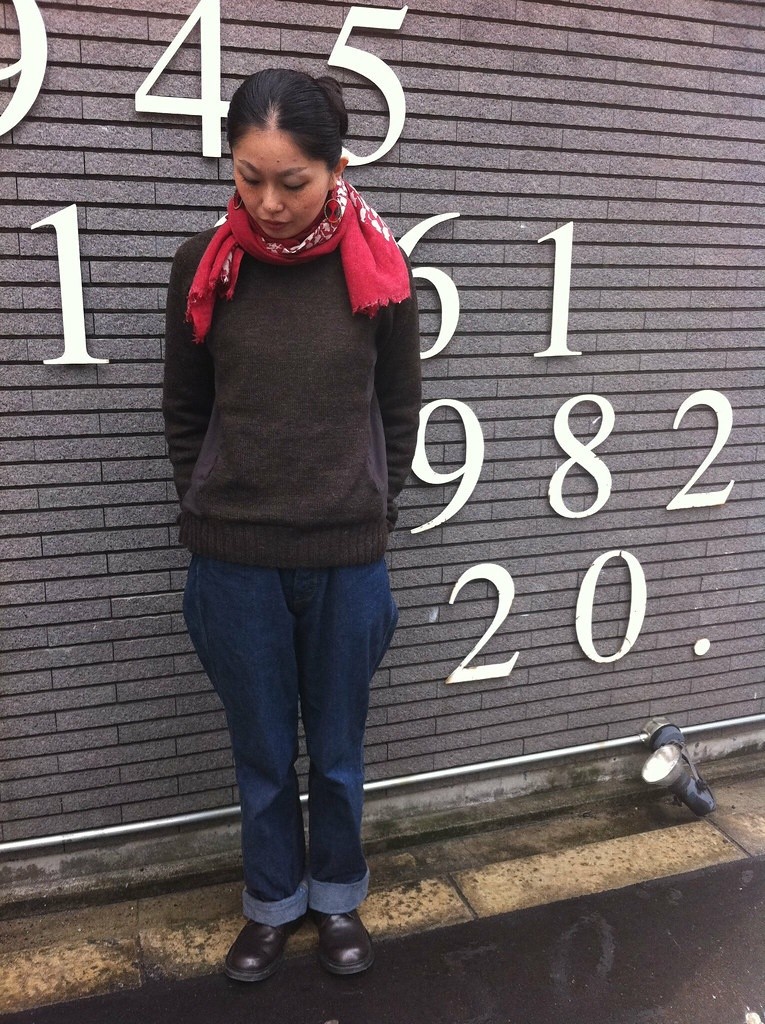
[641,724,716,817]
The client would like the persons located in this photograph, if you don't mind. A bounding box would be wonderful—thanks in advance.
[162,68,421,981]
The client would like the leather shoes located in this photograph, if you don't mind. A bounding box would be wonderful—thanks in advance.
[224,911,308,982]
[308,907,374,974]
